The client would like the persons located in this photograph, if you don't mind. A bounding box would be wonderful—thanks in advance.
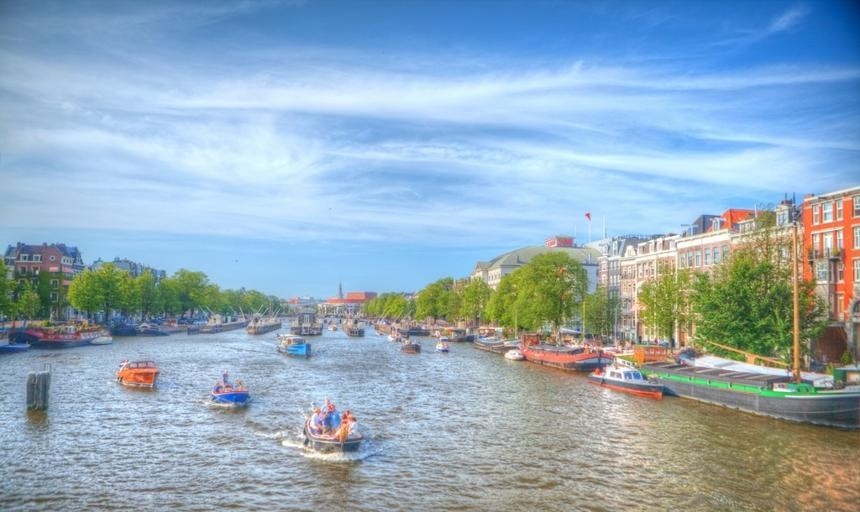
[211,368,245,397]
[311,402,360,436]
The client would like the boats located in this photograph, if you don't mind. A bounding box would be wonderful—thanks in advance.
[0,341,31,353]
[503,349,525,363]
[398,342,422,355]
[470,324,520,353]
[116,358,160,390]
[585,357,665,402]
[521,301,614,372]
[210,382,250,403]
[6,318,112,347]
[436,340,449,352]
[275,335,310,360]
[301,402,363,454]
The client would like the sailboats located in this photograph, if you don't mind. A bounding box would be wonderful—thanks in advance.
[628,196,860,436]
